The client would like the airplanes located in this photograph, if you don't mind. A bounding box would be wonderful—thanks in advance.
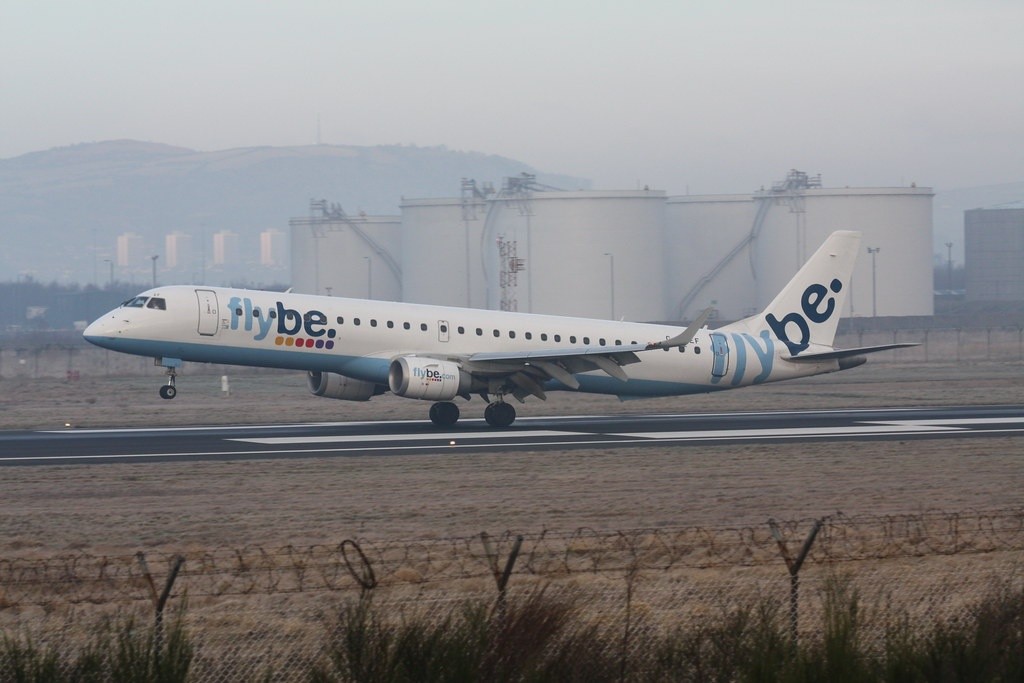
[78,226,917,428]
